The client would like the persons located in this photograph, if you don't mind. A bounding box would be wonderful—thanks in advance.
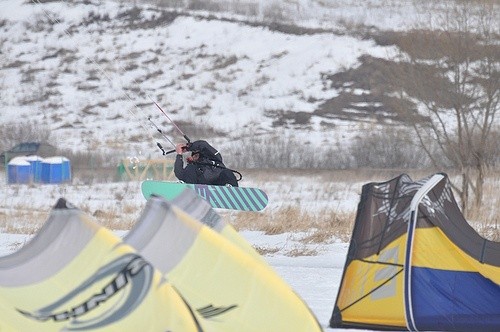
[174,140,238,188]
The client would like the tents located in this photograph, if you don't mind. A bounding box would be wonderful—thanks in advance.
[326,171,500,332]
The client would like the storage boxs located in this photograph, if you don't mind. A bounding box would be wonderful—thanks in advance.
[8,156,70,185]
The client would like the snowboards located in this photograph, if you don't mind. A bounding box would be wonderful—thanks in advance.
[142,180,267,211]
